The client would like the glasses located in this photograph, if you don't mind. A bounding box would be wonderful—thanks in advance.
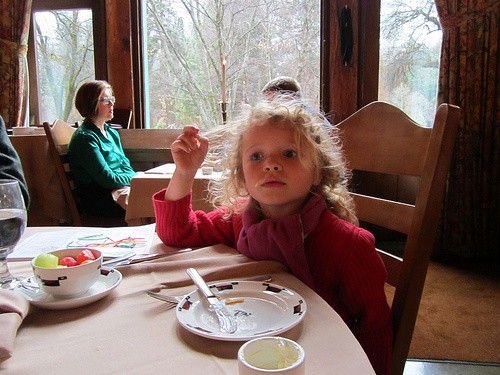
[97,96,115,105]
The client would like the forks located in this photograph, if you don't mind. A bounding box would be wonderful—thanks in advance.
[145,291,189,303]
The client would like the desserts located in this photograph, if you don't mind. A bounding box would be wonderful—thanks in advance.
[35,249,97,267]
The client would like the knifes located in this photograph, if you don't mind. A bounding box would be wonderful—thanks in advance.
[187,267,237,334]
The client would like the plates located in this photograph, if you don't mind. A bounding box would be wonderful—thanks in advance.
[16,265,123,309]
[175,280,308,341]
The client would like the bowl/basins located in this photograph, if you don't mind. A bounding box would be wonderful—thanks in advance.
[31,248,105,299]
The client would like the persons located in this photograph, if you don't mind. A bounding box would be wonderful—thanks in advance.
[0,115,30,247]
[152,76,392,375]
[68,81,137,218]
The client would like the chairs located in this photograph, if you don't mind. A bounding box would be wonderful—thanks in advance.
[315,101,461,375]
[42,118,128,226]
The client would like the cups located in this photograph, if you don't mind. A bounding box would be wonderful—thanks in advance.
[237,336,306,375]
[202,168,212,174]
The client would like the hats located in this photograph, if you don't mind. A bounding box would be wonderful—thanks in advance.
[260,77,302,97]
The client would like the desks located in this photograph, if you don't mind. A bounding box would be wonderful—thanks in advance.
[7,134,70,220]
[123,161,231,226]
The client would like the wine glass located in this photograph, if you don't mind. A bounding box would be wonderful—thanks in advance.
[0,179,28,293]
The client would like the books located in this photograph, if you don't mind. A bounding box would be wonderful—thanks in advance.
[106,108,133,129]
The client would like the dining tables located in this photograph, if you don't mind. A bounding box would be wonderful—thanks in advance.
[0,223,378,375]
